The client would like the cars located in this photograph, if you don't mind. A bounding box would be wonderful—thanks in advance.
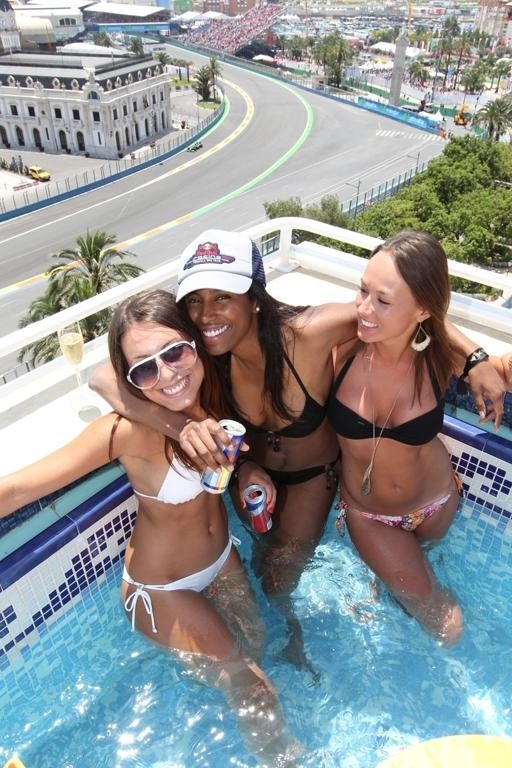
[27,166,53,183]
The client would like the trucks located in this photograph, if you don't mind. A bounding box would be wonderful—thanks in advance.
[400,103,433,113]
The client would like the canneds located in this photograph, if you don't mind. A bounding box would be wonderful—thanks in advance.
[243,484,273,534]
[200,419,247,495]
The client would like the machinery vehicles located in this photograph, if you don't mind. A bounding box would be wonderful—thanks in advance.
[453,104,471,126]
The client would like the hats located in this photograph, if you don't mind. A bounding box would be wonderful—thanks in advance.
[175,228,267,303]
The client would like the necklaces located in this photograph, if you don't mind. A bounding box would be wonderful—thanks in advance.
[360,352,416,497]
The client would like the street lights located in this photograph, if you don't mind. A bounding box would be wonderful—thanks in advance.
[195,87,201,126]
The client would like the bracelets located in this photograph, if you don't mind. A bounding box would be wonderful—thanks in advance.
[460,345,491,375]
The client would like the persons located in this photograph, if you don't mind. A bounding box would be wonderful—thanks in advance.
[86,229,506,682]
[1,289,306,768]
[324,230,511,649]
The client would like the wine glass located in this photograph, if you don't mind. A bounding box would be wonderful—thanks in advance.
[54,320,91,397]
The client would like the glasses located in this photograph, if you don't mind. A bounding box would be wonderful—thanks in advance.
[126,340,198,390]
